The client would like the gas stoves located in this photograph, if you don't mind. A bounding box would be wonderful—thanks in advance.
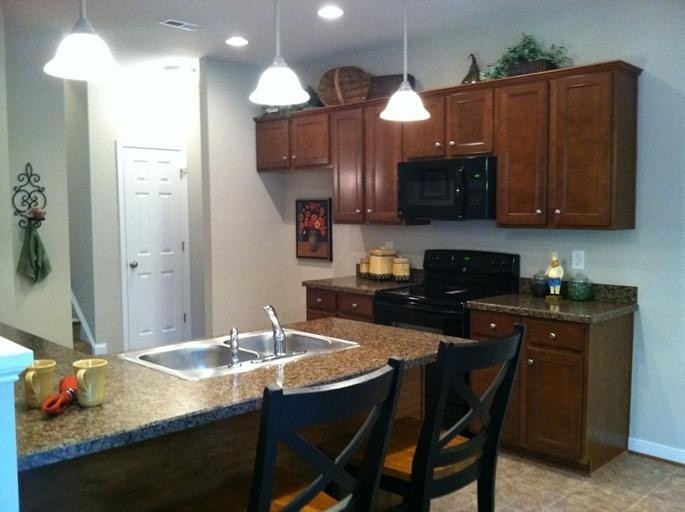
[376,279,511,303]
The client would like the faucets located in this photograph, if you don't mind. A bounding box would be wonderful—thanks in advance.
[262,304,286,355]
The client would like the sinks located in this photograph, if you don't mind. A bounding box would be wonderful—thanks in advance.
[117,339,259,383]
[222,327,361,366]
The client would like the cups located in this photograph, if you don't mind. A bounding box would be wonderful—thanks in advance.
[71,358,108,409]
[23,359,56,407]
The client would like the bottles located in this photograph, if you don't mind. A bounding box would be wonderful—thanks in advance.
[368,247,396,283]
[530,269,551,298]
[358,256,371,278]
[567,273,592,302]
[393,257,410,281]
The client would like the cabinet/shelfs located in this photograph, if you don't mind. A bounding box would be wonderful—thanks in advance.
[329,96,402,224]
[307,287,375,323]
[469,307,634,477]
[253,107,330,172]
[495,60,644,231]
[403,80,495,160]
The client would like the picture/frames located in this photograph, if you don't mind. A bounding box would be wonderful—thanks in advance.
[296,198,332,262]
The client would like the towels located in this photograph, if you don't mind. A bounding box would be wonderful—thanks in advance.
[16,222,53,285]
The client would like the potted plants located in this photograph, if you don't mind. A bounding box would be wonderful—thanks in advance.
[483,32,571,78]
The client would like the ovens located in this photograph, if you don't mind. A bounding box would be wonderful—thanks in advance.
[373,300,469,440]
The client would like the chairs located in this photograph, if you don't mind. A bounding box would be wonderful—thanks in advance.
[316,323,527,512]
[172,355,404,512]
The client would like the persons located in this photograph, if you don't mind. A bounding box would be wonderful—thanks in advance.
[545,250,565,296]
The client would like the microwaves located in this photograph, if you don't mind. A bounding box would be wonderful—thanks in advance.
[397,155,495,222]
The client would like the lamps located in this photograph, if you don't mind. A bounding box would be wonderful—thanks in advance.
[248,0,311,106]
[380,12,431,123]
[43,0,118,82]
